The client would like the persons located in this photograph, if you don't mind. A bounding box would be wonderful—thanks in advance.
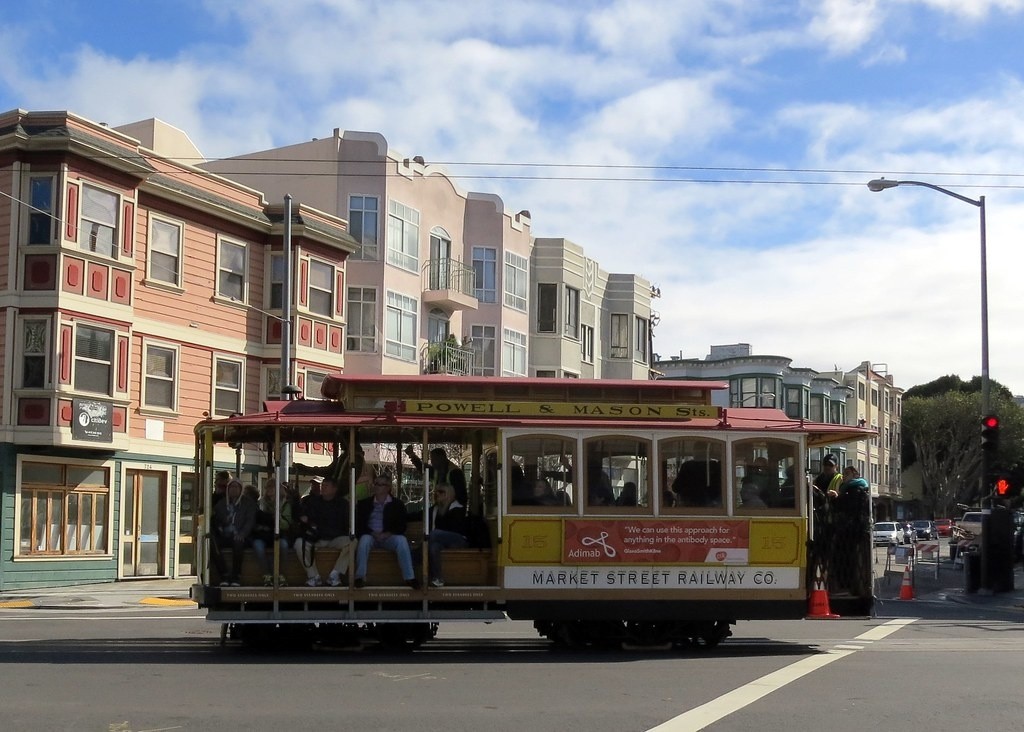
[405,445,468,510]
[422,483,470,587]
[355,475,422,591]
[212,470,292,587]
[540,455,636,507]
[294,478,358,586]
[662,441,722,508]
[300,476,325,504]
[740,454,870,596]
[292,440,364,497]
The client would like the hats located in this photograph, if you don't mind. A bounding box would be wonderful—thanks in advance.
[226,478,243,512]
[823,454,837,466]
[310,476,325,483]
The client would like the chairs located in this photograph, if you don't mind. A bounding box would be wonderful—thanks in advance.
[623,482,636,506]
[663,491,673,507]
[555,491,570,507]
[766,495,795,508]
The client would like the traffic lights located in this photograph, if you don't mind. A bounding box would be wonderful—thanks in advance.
[982,416,999,452]
[992,475,1016,498]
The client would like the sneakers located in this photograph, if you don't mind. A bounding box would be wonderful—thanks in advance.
[263,574,273,587]
[279,574,288,587]
[304,574,323,586]
[325,574,343,587]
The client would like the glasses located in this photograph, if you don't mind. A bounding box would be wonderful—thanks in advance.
[842,472,854,476]
[438,490,446,494]
[823,462,837,468]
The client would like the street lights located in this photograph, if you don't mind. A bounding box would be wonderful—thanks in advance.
[868,178,990,594]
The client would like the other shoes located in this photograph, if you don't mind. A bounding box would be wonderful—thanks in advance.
[220,576,229,586]
[430,577,444,586]
[354,578,368,588]
[406,579,421,590]
[230,573,241,586]
[831,588,849,595]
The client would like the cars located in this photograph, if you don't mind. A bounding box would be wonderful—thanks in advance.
[873,519,954,547]
[949,512,1024,561]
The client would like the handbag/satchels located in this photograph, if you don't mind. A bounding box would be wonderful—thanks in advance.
[300,523,318,542]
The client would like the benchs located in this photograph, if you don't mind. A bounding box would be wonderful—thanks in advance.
[218,546,496,585]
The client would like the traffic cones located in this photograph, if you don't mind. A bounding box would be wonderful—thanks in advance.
[807,565,840,620]
[895,566,918,600]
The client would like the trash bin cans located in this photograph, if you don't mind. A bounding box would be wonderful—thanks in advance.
[963,553,978,595]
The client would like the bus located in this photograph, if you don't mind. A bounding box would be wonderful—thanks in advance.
[189,375,880,659]
